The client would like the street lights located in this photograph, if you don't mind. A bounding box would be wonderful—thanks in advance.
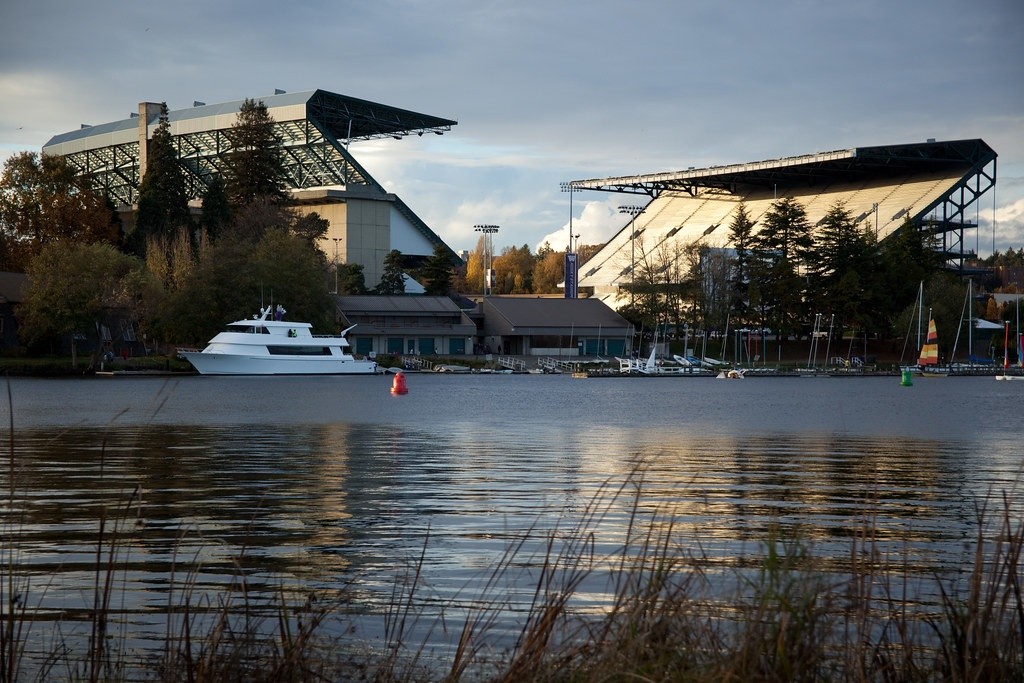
[474,225,499,296]
[333,238,342,293]
[618,206,647,307]
[559,182,585,254]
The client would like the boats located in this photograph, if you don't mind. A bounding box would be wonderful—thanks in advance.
[176,278,386,376]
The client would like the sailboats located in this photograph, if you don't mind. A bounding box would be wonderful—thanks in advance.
[948,277,993,371]
[916,318,947,377]
[995,320,1024,380]
[897,280,928,373]
[795,311,855,373]
[562,313,772,373]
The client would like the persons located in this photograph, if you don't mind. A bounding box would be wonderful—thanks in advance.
[288,329,297,337]
[121,349,128,360]
[105,349,114,363]
[844,358,850,372]
